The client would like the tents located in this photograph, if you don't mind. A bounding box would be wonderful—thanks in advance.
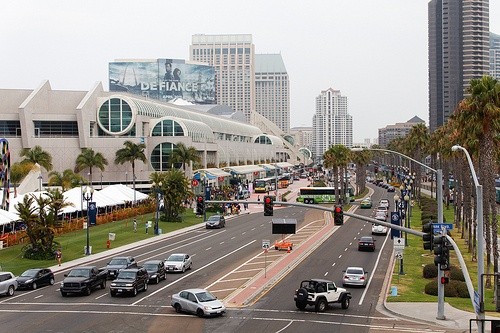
[100,185,148,208]
[0,186,115,234]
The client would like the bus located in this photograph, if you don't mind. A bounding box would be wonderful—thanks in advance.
[298,187,355,205]
[254,178,277,193]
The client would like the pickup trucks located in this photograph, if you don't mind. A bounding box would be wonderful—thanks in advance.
[59,265,109,297]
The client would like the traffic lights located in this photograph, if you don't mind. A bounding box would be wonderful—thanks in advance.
[433,236,449,266]
[196,195,205,216]
[422,221,432,251]
[333,205,343,226]
[263,195,274,216]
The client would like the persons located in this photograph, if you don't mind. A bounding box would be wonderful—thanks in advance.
[164,63,173,95]
[228,199,247,215]
[55,249,61,266]
[146,221,148,234]
[209,182,243,201]
[133,217,137,232]
[173,68,183,97]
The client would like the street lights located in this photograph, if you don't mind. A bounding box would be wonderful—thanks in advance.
[393,193,409,246]
[399,172,416,233]
[350,147,446,322]
[452,144,485,333]
[83,183,94,254]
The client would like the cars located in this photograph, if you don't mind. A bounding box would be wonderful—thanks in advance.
[367,179,395,193]
[206,214,225,229]
[360,197,372,208]
[371,199,389,235]
[106,256,138,281]
[15,269,54,291]
[163,253,193,274]
[357,236,377,251]
[142,260,167,284]
[341,267,369,287]
[109,266,149,297]
[170,288,226,318]
[0,271,18,297]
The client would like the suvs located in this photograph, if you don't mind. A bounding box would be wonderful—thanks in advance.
[294,278,351,311]
